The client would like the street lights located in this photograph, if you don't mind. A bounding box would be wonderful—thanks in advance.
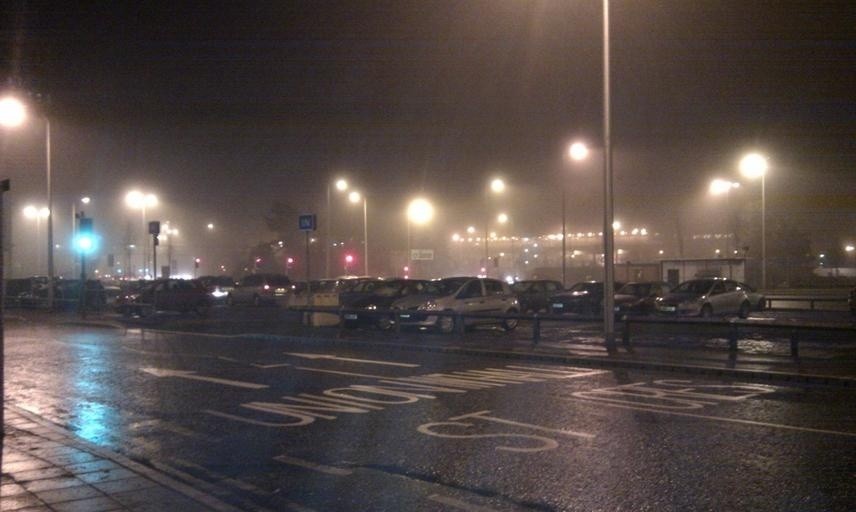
[740,151,770,297]
[349,190,368,276]
[0,93,181,275]
[484,177,507,278]
[709,179,746,239]
[561,140,590,286]
[326,178,349,278]
[498,212,517,283]
[407,198,435,281]
